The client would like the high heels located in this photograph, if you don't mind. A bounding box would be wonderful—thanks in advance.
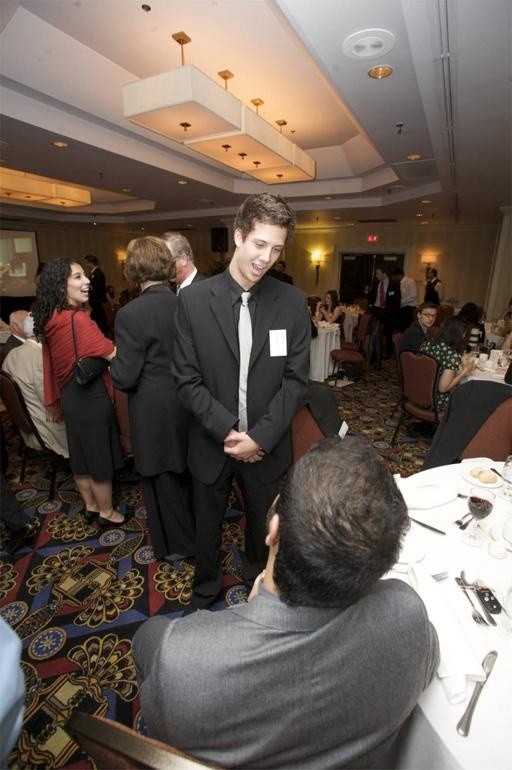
[97,511,132,532]
[84,510,99,525]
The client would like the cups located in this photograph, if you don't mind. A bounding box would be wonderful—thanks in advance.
[480,353,489,362]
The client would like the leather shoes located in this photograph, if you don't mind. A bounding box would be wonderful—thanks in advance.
[6,523,38,556]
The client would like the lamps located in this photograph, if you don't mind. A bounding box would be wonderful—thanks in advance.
[184,69,295,172]
[245,120,317,186]
[0,169,53,203]
[121,29,242,143]
[36,183,92,209]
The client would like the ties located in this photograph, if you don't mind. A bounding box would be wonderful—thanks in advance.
[237,290,253,431]
[379,282,384,309]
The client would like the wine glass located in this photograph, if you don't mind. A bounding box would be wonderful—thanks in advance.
[496,354,508,374]
[460,487,496,547]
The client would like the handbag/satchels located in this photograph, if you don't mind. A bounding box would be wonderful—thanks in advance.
[71,309,107,385]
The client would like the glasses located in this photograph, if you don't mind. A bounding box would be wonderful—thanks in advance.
[422,313,436,318]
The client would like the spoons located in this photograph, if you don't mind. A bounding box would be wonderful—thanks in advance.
[461,569,498,627]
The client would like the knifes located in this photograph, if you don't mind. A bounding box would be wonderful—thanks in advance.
[454,650,499,737]
[408,516,446,535]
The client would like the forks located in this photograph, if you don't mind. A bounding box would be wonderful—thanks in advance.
[455,576,489,628]
[386,567,450,586]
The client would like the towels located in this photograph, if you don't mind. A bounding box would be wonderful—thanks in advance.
[436,660,484,705]
[393,473,432,510]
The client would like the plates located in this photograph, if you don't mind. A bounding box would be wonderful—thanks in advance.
[461,466,504,488]
[477,363,495,373]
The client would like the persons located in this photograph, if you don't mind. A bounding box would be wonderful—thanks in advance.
[428,305,455,337]
[4,311,29,363]
[107,236,193,563]
[368,265,400,358]
[1,334,69,462]
[392,267,416,332]
[400,302,438,352]
[34,262,47,282]
[31,257,132,532]
[502,311,511,351]
[424,268,444,306]
[0,418,38,556]
[317,290,344,345]
[308,305,318,338]
[84,255,109,337]
[267,263,294,285]
[461,303,485,351]
[277,260,286,271]
[106,285,116,311]
[169,191,311,617]
[133,435,438,770]
[162,231,208,297]
[416,315,476,412]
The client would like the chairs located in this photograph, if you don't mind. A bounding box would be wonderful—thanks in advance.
[0,370,70,500]
[63,710,220,770]
[422,379,512,472]
[331,314,372,391]
[391,349,445,446]
[390,330,405,421]
[276,384,341,475]
